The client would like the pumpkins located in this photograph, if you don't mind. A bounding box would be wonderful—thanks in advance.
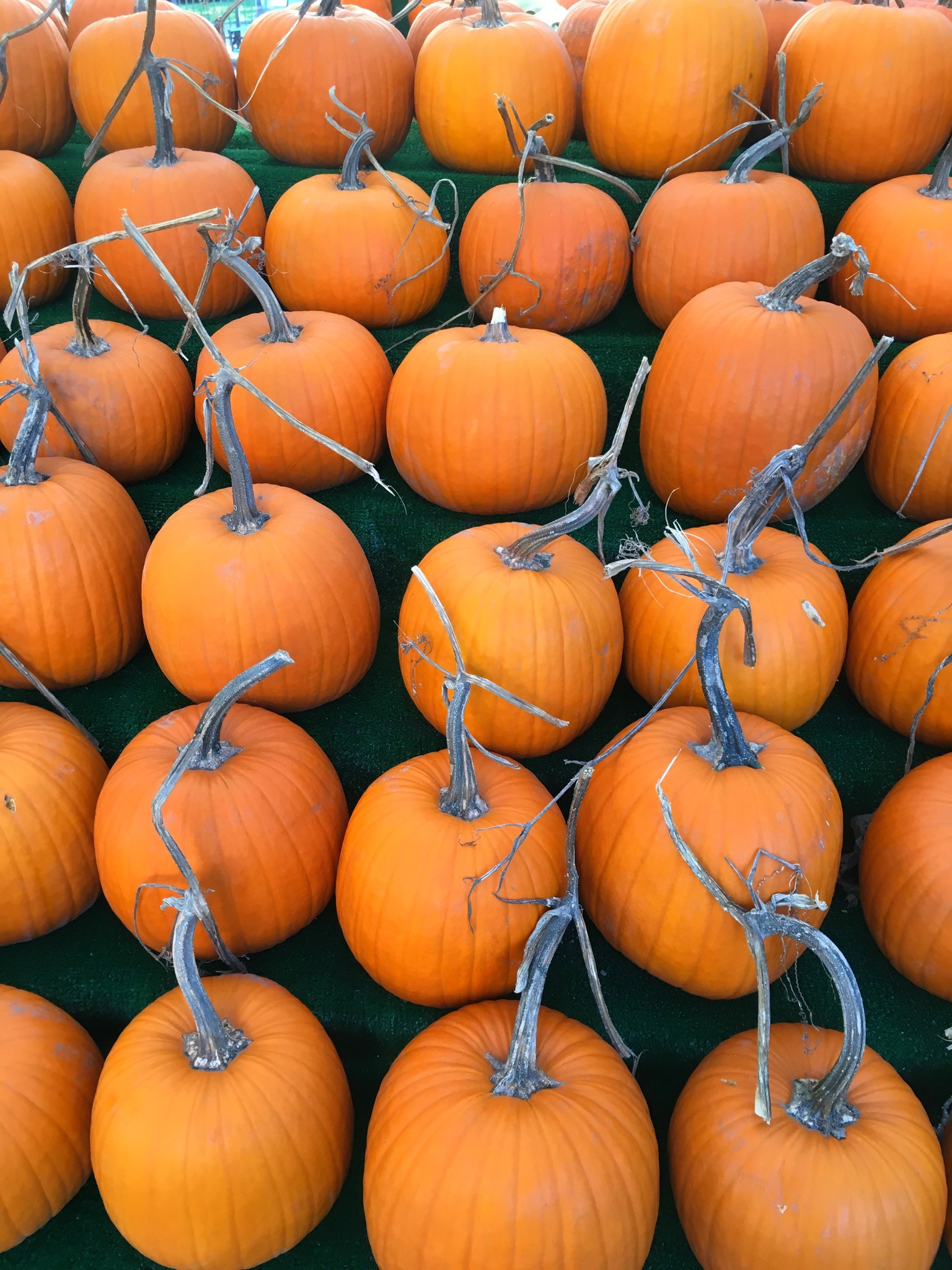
[2,2,951,1270]
